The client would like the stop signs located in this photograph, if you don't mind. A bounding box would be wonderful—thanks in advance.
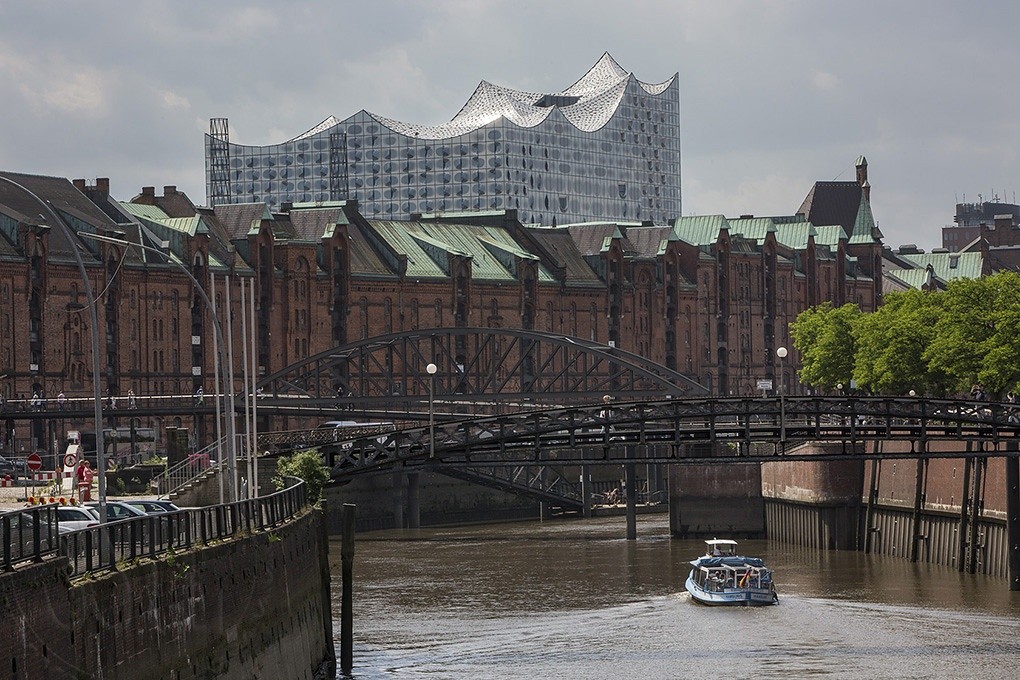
[27,454,42,470]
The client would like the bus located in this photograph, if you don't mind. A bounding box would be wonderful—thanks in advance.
[311,421,396,450]
[81,427,156,466]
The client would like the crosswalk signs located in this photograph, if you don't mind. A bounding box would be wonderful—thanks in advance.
[63,454,77,467]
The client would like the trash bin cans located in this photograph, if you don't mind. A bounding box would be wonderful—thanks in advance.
[78,482,90,501]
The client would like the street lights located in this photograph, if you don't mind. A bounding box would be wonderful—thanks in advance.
[426,363,439,458]
[777,347,788,441]
[77,232,235,501]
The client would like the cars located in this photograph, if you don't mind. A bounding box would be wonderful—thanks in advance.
[0,508,83,569]
[24,506,100,549]
[113,500,185,533]
[15,462,31,479]
[84,502,178,546]
[0,455,18,480]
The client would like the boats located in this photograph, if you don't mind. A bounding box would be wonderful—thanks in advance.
[592,501,667,516]
[685,540,779,606]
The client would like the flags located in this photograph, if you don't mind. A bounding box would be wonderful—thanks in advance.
[740,569,750,588]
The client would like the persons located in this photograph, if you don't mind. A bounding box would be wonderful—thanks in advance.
[75,459,94,501]
[971,383,1019,424]
[103,388,137,411]
[599,395,617,440]
[194,385,206,407]
[58,388,66,411]
[336,386,356,410]
[0,388,45,414]
[706,545,733,588]
